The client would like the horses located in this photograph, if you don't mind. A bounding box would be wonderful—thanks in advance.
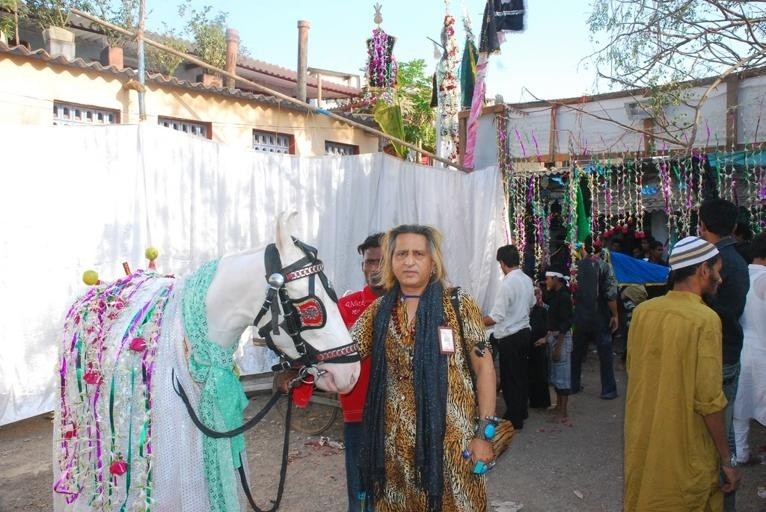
[61,210,361,512]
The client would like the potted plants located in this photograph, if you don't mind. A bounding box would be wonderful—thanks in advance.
[42,0,147,69]
[182,0,228,85]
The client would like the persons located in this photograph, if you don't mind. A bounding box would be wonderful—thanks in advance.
[733,206,754,266]
[483,244,536,431]
[611,236,669,299]
[348,225,499,511]
[571,233,620,400]
[621,236,739,512]
[732,231,766,464]
[272,232,387,512]
[534,265,575,424]
[698,198,749,512]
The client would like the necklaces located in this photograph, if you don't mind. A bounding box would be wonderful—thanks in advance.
[403,294,422,299]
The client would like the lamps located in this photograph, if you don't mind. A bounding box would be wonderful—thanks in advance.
[623,98,656,120]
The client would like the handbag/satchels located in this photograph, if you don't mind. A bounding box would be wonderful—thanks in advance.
[466,417,515,475]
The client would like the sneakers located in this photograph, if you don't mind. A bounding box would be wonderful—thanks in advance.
[602,391,618,398]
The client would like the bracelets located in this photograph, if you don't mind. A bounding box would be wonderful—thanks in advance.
[721,460,738,468]
[475,419,495,441]
[474,413,498,422]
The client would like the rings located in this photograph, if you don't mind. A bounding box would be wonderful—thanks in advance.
[470,460,496,475]
[463,448,469,458]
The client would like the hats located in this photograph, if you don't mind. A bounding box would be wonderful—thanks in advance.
[669,235,721,271]
